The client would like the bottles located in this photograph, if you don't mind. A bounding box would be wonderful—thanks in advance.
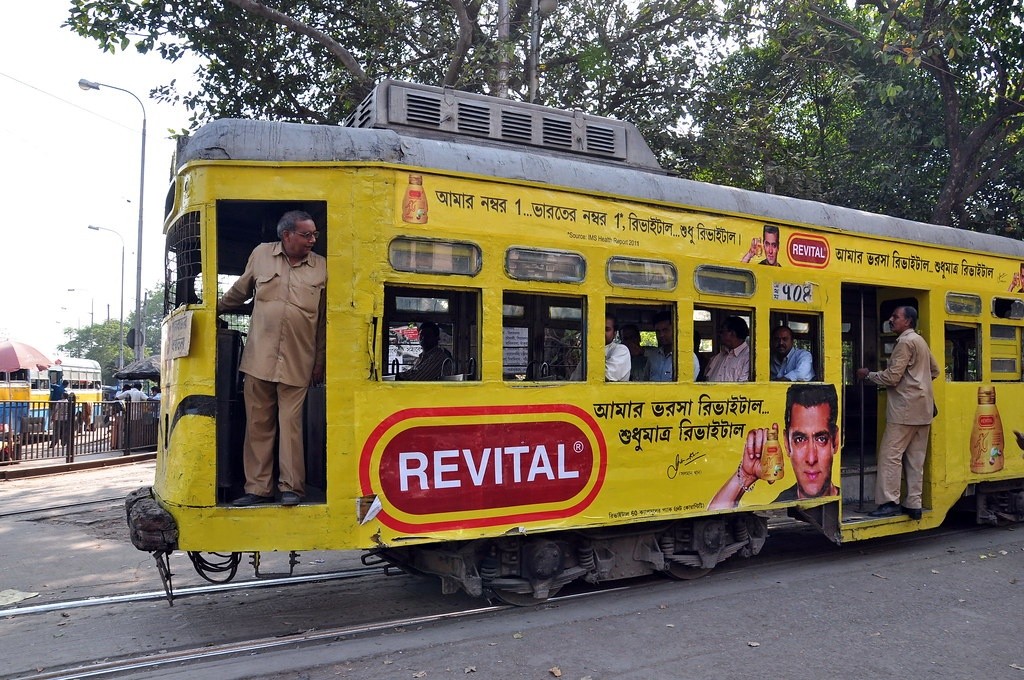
[1016,275,1020,287]
[761,429,785,480]
[402,174,429,224]
[970,386,1005,473]
[756,239,762,257]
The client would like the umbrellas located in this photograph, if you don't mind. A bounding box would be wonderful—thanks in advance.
[113,354,161,387]
[0,339,52,400]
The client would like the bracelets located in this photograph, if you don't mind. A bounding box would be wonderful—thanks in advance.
[736,459,757,493]
[749,250,752,258]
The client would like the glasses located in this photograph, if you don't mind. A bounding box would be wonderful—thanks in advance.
[289,231,319,239]
[621,335,636,341]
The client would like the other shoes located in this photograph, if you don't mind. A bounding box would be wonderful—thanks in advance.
[233,494,275,505]
[281,491,300,505]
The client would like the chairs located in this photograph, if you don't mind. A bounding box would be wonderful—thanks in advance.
[523,360,559,381]
[392,358,477,382]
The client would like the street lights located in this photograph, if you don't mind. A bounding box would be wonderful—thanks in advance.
[66,288,94,349]
[60,305,82,358]
[76,78,147,367]
[87,225,125,393]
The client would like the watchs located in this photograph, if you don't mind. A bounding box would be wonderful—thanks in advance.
[865,371,872,382]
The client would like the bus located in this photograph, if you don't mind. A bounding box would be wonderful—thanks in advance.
[0,354,105,442]
[123,80,1024,607]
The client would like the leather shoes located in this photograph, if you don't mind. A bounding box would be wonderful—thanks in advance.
[868,505,900,516]
[901,505,921,520]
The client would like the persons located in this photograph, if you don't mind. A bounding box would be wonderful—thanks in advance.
[1008,263,1024,293]
[219,210,327,506]
[704,316,749,383]
[707,383,840,510]
[856,306,940,520]
[48,393,68,448]
[395,320,452,381]
[50,380,69,411]
[742,224,782,267]
[570,314,701,383]
[770,325,816,382]
[111,383,162,452]
[50,358,63,386]
[95,381,100,389]
